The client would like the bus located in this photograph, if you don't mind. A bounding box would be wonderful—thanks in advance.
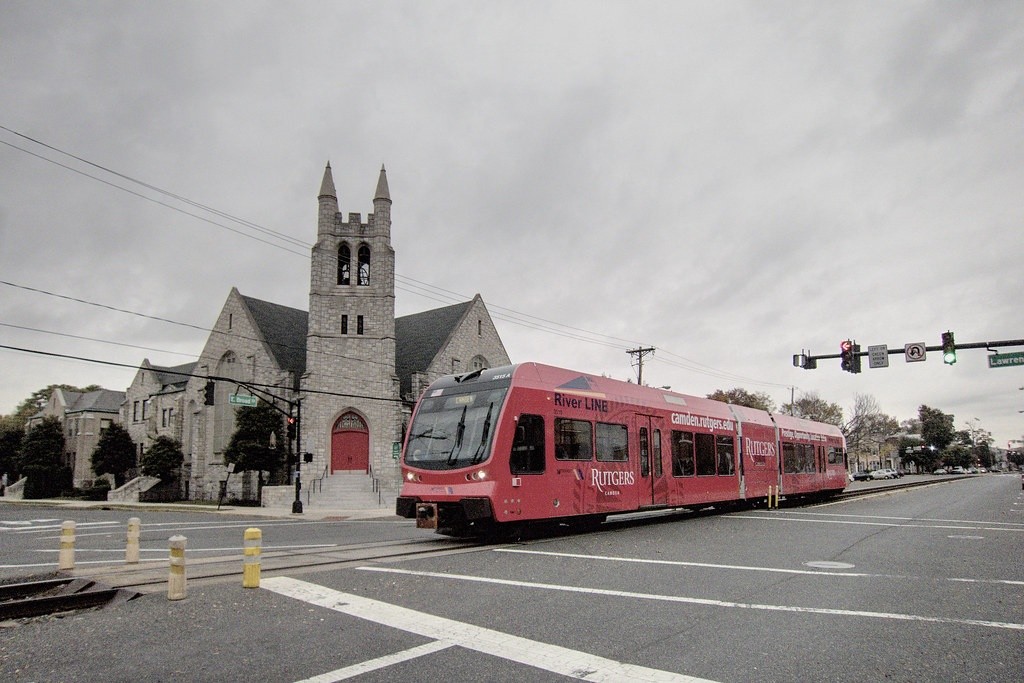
[390,360,851,539]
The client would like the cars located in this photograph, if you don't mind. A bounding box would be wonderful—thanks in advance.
[868,471,893,480]
[934,469,948,475]
[847,474,855,485]
[952,466,987,474]
[851,472,874,482]
[877,468,904,479]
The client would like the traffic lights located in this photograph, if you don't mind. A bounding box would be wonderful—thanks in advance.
[305,453,314,462]
[285,417,297,439]
[942,331,956,364]
[840,341,855,372]
